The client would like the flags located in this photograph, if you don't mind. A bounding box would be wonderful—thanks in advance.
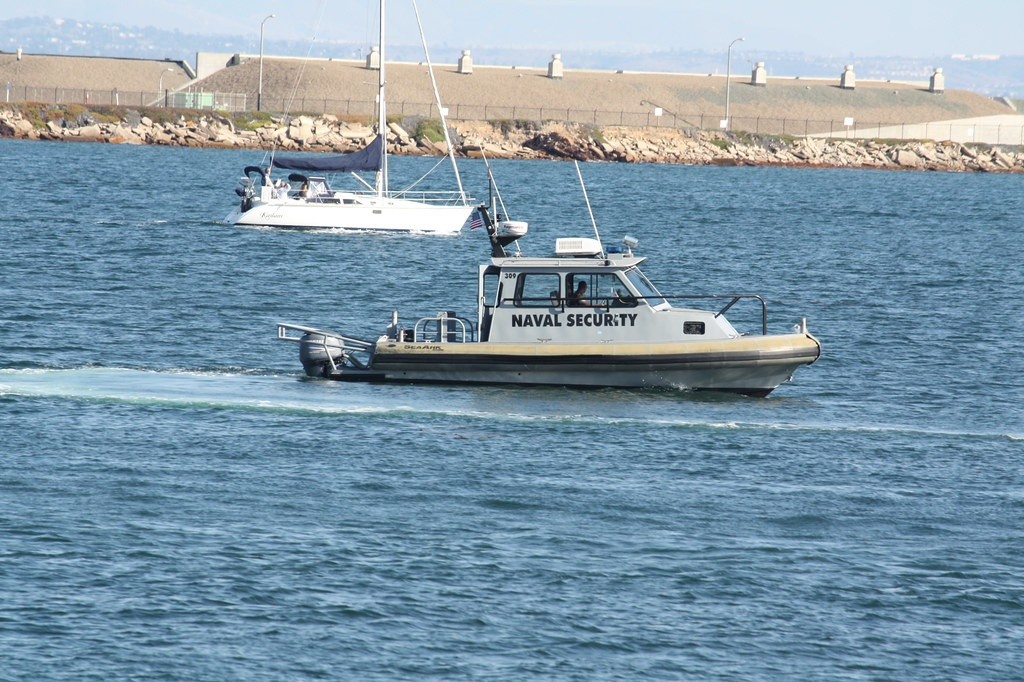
[469,212,484,231]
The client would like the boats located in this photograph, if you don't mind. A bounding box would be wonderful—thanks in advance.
[277,142,821,399]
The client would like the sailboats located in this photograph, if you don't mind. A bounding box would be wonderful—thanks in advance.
[223,0,475,238]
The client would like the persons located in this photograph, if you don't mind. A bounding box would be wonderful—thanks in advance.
[566,281,604,306]
[260,167,291,201]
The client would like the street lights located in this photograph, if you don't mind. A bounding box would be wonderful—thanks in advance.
[257,12,274,113]
[724,38,746,127]
